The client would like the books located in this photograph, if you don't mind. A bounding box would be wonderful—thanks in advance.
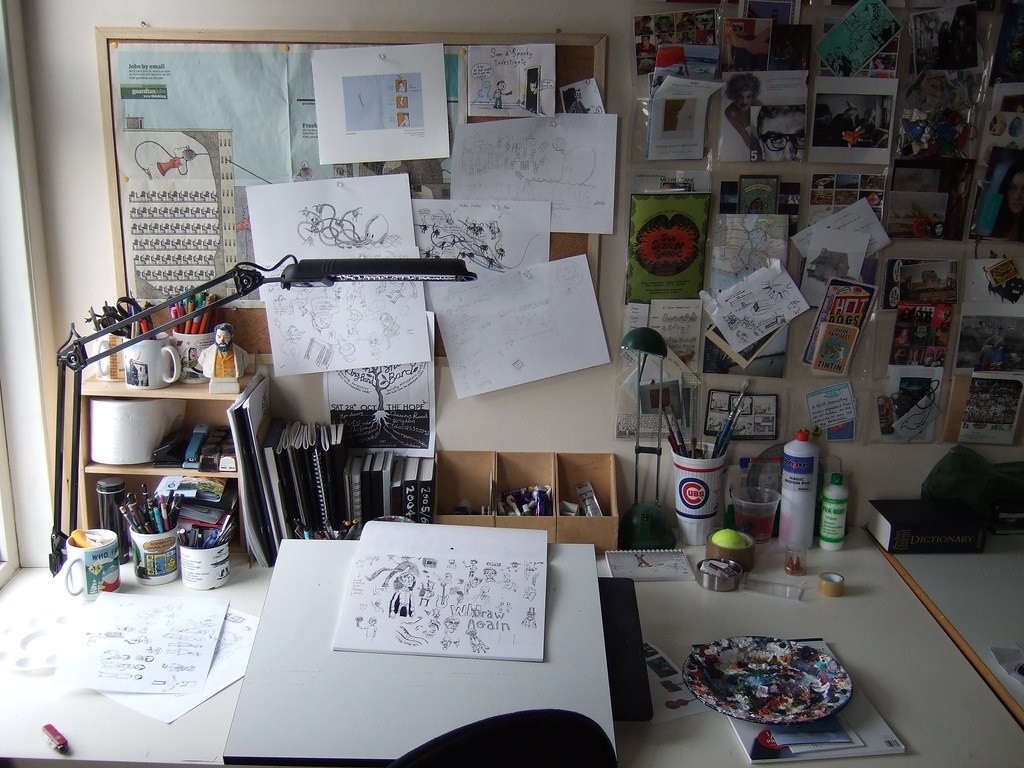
[690,637,906,764]
[864,497,985,554]
[985,500,1024,535]
[226,370,435,569]
[154,475,238,531]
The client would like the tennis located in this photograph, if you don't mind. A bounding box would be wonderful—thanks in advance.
[710,529,747,548]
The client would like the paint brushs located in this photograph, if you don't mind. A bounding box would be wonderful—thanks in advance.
[659,378,753,457]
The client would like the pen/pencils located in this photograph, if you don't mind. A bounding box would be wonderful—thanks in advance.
[119,484,181,533]
[182,521,237,545]
[302,520,357,538]
[129,291,155,340]
[166,288,219,332]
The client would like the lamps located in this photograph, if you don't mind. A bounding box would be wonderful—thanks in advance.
[618,327,677,550]
[49,254,477,576]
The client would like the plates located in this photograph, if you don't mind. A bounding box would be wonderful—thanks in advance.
[683,637,853,725]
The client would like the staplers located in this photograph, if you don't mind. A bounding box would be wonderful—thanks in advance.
[182,422,210,469]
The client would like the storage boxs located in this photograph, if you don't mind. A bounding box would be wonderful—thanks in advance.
[435,450,619,554]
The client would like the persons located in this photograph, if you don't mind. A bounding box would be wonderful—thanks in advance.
[990,160,1024,241]
[756,105,806,162]
[635,13,716,73]
[724,73,768,149]
[937,18,974,67]
[197,323,251,379]
[893,307,951,367]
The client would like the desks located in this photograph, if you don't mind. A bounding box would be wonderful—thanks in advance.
[0,521,1024,768]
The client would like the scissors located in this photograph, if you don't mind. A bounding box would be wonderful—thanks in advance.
[101,296,142,335]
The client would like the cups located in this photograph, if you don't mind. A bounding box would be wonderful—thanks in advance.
[178,529,230,590]
[123,334,181,390]
[130,525,178,586]
[62,529,121,601]
[784,542,807,576]
[732,485,781,545]
[93,331,125,382]
[671,444,725,546]
[173,328,215,383]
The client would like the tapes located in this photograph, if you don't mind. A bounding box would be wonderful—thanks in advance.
[818,572,843,596]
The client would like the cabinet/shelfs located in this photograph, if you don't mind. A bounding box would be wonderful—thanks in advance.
[80,373,255,552]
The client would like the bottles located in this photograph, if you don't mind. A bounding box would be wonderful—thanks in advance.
[780,429,820,549]
[820,473,848,550]
